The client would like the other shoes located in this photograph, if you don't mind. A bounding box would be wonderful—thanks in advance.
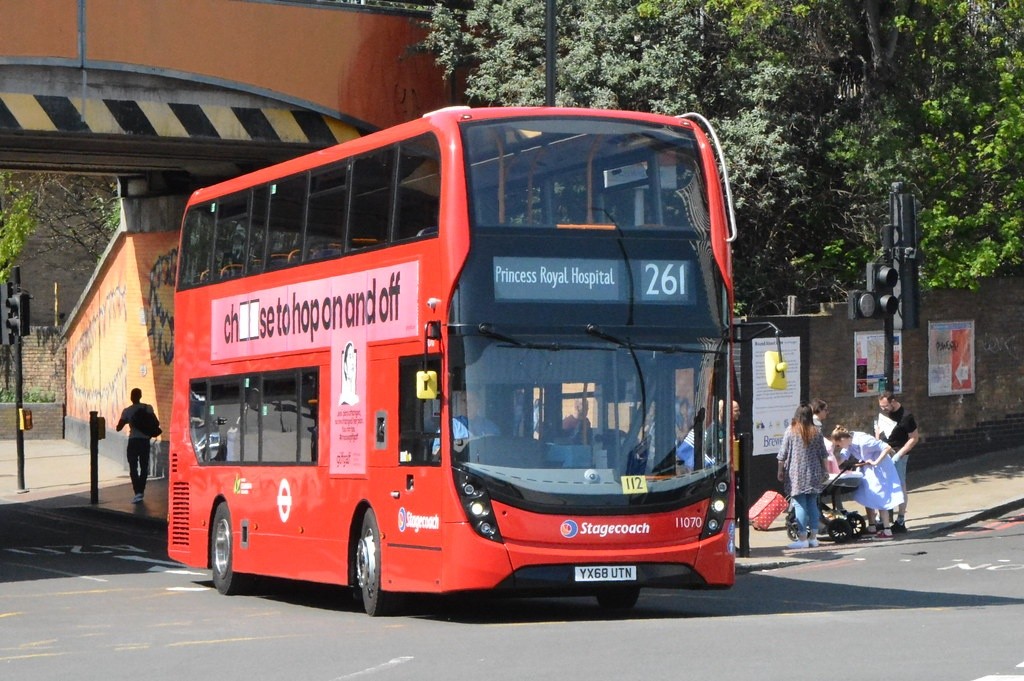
[128,493,144,503]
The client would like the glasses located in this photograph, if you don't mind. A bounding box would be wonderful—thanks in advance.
[821,408,827,413]
[835,444,839,449]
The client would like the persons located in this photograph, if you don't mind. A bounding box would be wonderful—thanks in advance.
[873,389,919,532]
[432,385,501,458]
[776,403,828,548]
[637,399,740,469]
[813,398,828,432]
[562,397,591,429]
[512,390,544,439]
[831,426,895,540]
[116,389,159,505]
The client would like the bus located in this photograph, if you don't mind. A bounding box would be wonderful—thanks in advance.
[167,101,789,618]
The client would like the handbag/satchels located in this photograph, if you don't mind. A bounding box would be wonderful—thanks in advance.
[131,406,162,437]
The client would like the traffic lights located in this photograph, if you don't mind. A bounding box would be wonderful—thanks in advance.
[851,261,897,320]
[6,293,31,337]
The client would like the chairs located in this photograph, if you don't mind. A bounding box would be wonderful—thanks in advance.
[192,226,438,287]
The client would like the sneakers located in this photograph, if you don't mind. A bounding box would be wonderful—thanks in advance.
[890,521,907,533]
[808,539,819,547]
[876,523,884,531]
[872,532,894,540]
[787,539,809,548]
[861,527,877,537]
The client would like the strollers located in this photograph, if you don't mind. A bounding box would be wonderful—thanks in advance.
[785,459,866,542]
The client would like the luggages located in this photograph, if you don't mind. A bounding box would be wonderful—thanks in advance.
[748,491,791,531]
